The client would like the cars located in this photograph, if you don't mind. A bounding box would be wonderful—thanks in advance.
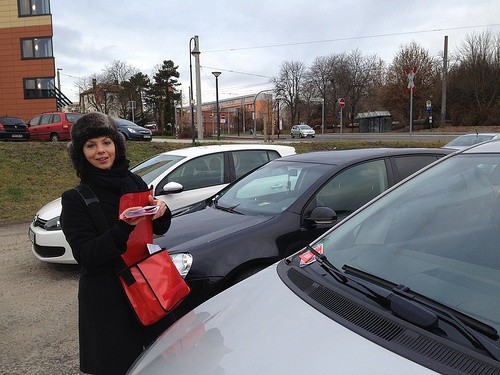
[126,132,500,375]
[290,125,316,138]
[0,117,30,141]
[113,118,152,142]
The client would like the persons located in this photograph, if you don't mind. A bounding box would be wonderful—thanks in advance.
[59,113,173,375]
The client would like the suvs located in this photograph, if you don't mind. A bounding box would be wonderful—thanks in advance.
[25,112,82,142]
[29,143,304,269]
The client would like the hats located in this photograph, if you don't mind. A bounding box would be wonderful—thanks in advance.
[66,112,126,168]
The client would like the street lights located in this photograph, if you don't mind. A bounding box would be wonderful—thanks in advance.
[253,89,278,138]
[189,37,201,141]
[236,107,240,136]
[211,72,222,140]
[56,68,64,112]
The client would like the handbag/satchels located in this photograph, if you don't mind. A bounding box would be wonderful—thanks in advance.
[117,185,191,327]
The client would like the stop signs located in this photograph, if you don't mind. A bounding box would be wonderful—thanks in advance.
[338,98,346,108]
[221,114,226,119]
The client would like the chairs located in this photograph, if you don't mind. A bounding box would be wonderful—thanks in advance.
[195,161,209,177]
[209,158,221,177]
